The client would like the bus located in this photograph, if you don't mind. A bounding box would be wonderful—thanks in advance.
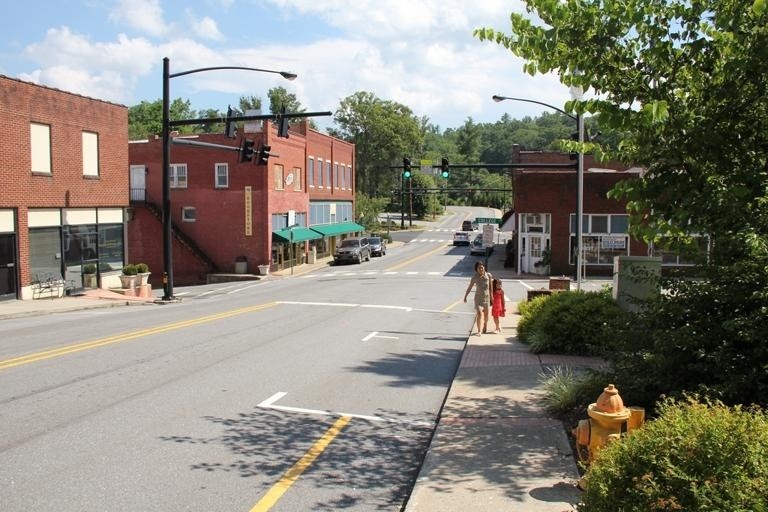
[378,213,407,226]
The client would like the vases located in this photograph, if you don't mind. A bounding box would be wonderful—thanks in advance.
[257,264,270,274]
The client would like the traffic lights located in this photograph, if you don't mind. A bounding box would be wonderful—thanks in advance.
[225,103,290,165]
[442,157,449,178]
[403,157,411,179]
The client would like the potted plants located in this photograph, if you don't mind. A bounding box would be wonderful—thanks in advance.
[120,264,138,288]
[135,263,151,286]
[83,264,96,286]
[99,264,112,272]
[535,250,551,275]
[234,256,248,273]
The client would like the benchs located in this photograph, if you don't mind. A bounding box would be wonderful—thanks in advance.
[37,272,76,299]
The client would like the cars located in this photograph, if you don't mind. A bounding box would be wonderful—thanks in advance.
[333,237,386,264]
[462,221,479,231]
[453,232,494,256]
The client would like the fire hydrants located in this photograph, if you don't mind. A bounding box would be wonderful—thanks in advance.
[571,384,646,493]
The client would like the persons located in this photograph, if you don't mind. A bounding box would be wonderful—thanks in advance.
[464,260,494,336]
[490,278,507,333]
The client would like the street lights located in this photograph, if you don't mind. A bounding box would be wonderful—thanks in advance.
[493,95,584,290]
[163,57,297,300]
